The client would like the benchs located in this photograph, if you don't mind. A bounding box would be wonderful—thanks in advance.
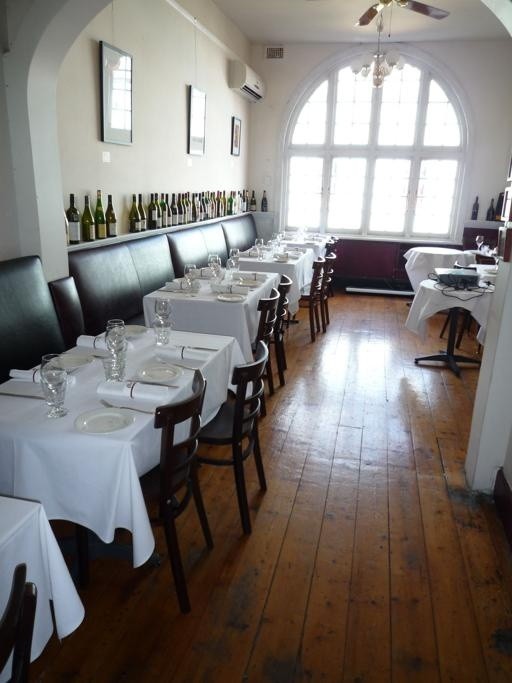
[69,213,261,338]
[1,255,69,381]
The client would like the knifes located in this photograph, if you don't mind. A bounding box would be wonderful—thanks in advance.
[157,288,181,293]
[0,393,45,400]
[174,345,218,352]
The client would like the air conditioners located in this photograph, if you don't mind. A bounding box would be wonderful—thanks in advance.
[229,59,264,103]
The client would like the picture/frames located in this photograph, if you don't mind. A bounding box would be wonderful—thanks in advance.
[496,160,512,261]
[230,117,242,158]
[187,84,206,159]
[100,40,132,147]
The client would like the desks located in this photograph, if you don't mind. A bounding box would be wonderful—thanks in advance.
[403,240,495,377]
[0,495,86,683]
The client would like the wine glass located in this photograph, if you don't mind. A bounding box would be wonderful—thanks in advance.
[184,248,240,291]
[40,353,68,418]
[155,296,173,345]
[255,230,285,262]
[105,319,125,364]
[475,235,485,251]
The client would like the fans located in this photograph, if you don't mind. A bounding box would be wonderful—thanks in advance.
[356,0,451,26]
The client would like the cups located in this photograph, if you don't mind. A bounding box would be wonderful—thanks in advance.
[481,244,489,254]
[156,322,171,345]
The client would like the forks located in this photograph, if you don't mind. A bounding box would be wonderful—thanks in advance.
[99,399,153,415]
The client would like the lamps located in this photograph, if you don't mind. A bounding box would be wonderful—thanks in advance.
[350,13,405,85]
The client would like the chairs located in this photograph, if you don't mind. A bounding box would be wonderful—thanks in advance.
[251,286,285,393]
[0,560,36,683]
[276,273,292,372]
[299,234,341,343]
[197,340,268,539]
[74,370,213,616]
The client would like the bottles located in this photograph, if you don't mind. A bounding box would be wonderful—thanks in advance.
[128,189,257,232]
[261,190,268,212]
[471,196,495,221]
[64,190,118,247]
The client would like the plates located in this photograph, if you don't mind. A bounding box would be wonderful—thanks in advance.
[51,325,182,434]
[218,279,262,302]
[172,277,186,282]
[484,270,498,274]
